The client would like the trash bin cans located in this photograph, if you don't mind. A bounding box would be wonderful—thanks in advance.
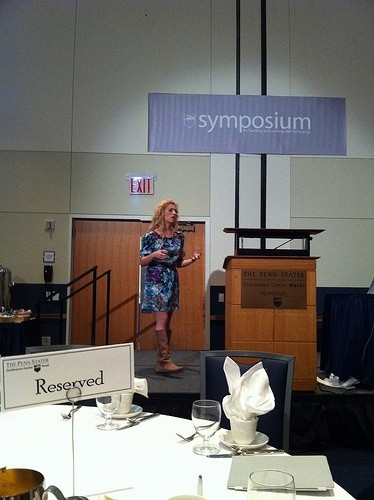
[0,309,32,358]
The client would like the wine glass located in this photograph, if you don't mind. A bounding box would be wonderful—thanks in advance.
[96,394,121,430]
[191,400,222,455]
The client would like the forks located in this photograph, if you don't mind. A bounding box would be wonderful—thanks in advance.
[175,432,199,442]
[60,405,81,419]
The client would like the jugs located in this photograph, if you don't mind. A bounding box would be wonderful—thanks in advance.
[0,466,66,500]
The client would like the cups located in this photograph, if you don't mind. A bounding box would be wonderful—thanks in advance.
[330,376,339,386]
[231,417,258,444]
[247,470,296,500]
[168,494,206,500]
[119,392,134,414]
[42,336,51,346]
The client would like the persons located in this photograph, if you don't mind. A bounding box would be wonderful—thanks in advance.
[139,198,202,374]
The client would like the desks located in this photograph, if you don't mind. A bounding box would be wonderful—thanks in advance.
[324,291,374,391]
[0,318,39,357]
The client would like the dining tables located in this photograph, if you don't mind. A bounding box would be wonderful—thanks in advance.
[0,406,358,500]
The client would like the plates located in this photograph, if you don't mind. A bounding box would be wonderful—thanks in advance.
[220,431,269,450]
[112,405,142,419]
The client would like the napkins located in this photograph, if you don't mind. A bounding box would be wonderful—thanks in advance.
[222,357,276,421]
[130,372,154,400]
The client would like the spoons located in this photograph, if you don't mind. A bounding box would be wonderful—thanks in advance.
[127,412,159,424]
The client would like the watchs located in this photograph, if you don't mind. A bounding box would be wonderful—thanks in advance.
[191,257,196,263]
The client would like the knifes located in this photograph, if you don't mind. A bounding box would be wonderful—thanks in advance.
[117,413,159,430]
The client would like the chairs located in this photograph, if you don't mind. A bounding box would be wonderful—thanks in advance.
[198,347,297,455]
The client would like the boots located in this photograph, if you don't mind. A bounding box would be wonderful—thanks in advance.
[154,329,185,373]
